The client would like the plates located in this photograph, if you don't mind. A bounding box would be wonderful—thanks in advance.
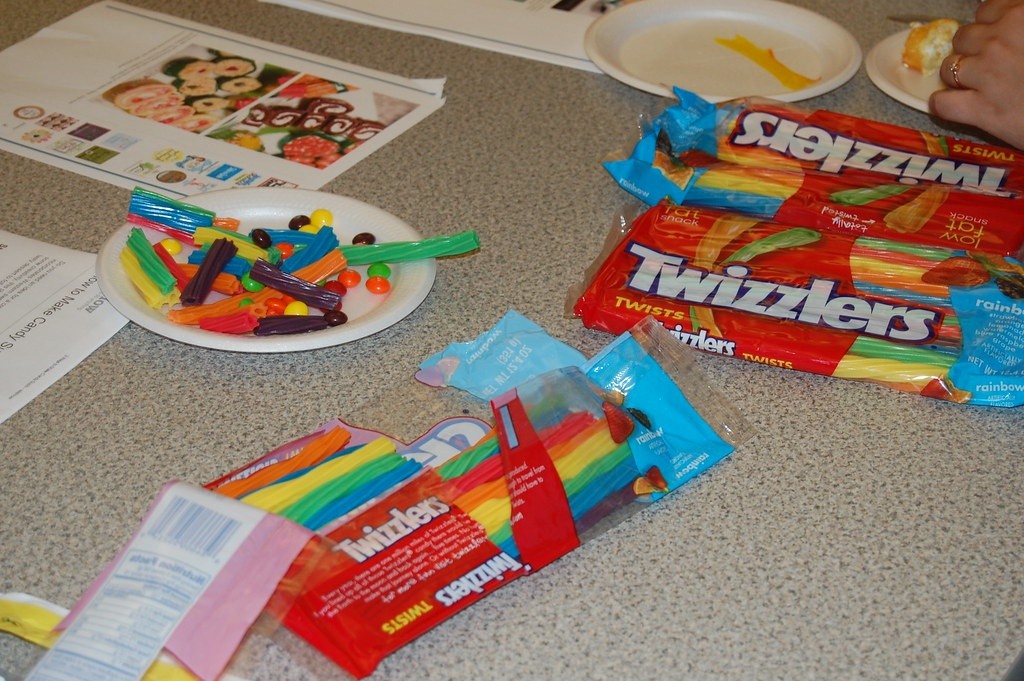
[865,27,950,116]
[582,0,864,104]
[94,185,437,354]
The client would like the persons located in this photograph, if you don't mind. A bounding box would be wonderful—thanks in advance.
[929,0,1024,152]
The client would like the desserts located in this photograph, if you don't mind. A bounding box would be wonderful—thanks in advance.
[901,18,964,77]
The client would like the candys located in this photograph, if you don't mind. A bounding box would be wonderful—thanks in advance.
[122,188,479,336]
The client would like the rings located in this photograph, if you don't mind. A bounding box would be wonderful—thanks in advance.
[950,56,964,87]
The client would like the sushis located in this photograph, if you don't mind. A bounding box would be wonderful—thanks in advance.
[114,55,385,170]
